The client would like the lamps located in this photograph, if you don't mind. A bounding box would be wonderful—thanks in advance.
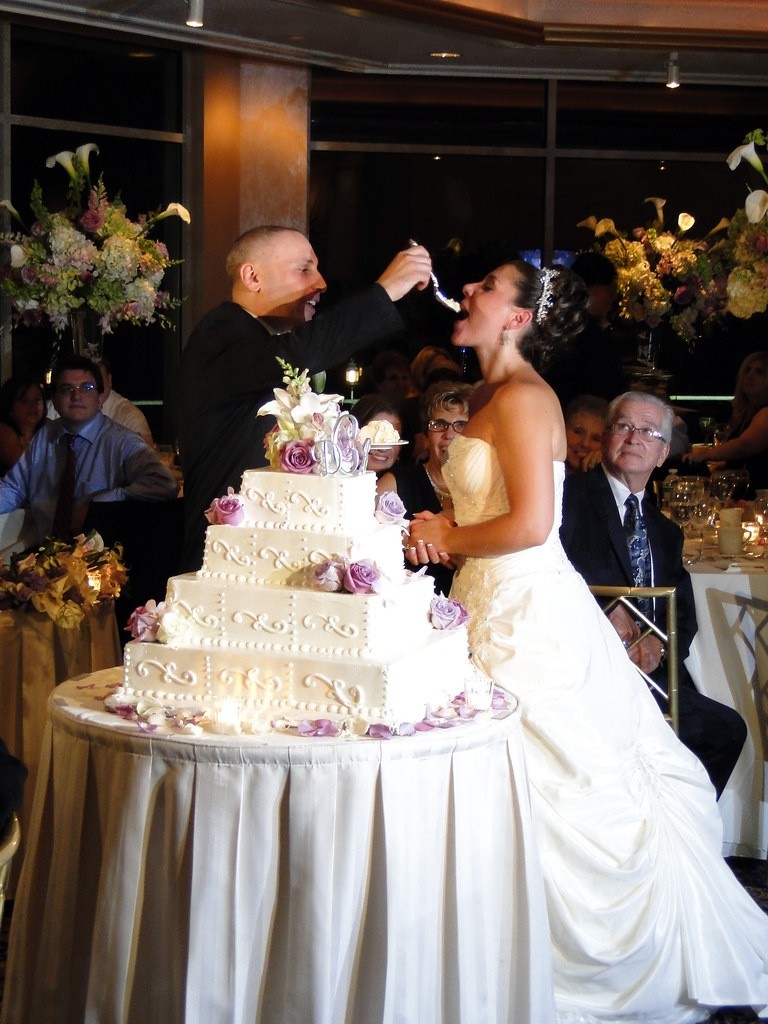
[183,0,204,27]
[664,61,681,88]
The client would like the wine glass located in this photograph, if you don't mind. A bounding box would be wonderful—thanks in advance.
[700,417,714,446]
[671,473,736,567]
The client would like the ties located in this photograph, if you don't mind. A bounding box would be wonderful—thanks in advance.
[50,434,80,542]
[624,493,654,626]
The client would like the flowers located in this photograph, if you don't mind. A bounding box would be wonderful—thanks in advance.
[203,487,245,526]
[374,491,408,524]
[257,357,364,474]
[0,531,128,629]
[0,142,191,335]
[431,590,469,629]
[312,554,387,595]
[576,197,730,343]
[125,597,189,645]
[553,249,576,270]
[521,249,541,269]
[726,126,768,320]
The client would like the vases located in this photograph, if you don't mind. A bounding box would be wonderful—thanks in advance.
[638,323,659,369]
[68,310,105,359]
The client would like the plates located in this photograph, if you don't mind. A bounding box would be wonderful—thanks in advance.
[362,440,410,450]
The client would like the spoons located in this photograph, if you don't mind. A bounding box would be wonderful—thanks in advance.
[408,238,460,313]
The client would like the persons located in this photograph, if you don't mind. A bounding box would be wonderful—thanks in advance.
[558,390,748,807]
[175,225,433,575]
[327,342,482,596]
[558,349,768,488]
[404,260,667,1024]
[0,350,197,662]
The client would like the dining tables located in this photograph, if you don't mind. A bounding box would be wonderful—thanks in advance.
[683,555,768,860]
[0,606,124,775]
[0,666,551,1024]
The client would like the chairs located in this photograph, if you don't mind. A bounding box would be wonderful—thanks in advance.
[84,497,185,650]
[585,585,679,738]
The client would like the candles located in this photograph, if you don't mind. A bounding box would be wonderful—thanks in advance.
[744,526,758,541]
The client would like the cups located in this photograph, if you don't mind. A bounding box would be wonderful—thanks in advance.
[711,508,744,530]
[717,527,753,556]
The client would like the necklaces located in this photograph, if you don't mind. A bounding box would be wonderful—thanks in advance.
[424,466,453,501]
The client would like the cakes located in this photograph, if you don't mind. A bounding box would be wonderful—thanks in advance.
[106,354,472,741]
[444,298,461,313]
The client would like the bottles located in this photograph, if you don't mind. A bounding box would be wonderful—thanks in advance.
[663,469,680,510]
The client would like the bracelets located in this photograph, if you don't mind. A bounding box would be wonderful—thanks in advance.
[660,643,667,661]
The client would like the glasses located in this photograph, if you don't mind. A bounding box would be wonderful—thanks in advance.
[53,382,98,394]
[608,423,668,445]
[427,419,469,434]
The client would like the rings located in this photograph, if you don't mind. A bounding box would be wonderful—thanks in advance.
[624,639,630,648]
